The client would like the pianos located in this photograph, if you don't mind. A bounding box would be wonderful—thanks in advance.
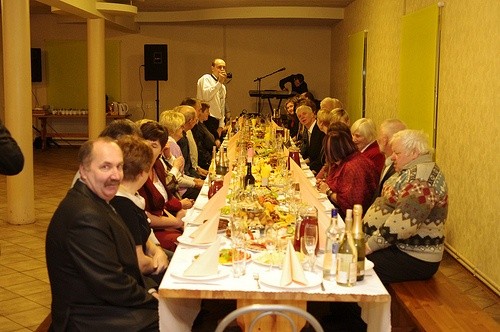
[249,89,299,116]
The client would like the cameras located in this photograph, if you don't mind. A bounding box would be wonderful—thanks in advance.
[226,73,232,78]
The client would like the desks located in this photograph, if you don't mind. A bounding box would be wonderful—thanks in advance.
[33,114,133,152]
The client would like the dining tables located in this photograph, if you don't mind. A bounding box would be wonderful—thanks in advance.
[157,116,392,332]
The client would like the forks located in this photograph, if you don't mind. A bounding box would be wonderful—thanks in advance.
[253,273,261,290]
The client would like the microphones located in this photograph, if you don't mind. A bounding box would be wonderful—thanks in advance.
[280,68,285,71]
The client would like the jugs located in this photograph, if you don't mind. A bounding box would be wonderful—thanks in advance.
[286,147,301,170]
[276,126,284,139]
[207,174,224,199]
[232,120,236,128]
[274,114,282,126]
[293,204,320,255]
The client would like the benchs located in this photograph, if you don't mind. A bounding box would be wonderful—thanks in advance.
[390,250,500,332]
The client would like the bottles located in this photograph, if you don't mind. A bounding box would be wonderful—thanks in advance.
[324,208,343,281]
[215,146,229,176]
[336,209,357,287]
[351,204,366,281]
[244,162,255,190]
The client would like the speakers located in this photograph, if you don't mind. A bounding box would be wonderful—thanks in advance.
[144,44,168,81]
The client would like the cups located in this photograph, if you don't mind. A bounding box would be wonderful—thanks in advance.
[300,236,314,257]
[232,247,246,278]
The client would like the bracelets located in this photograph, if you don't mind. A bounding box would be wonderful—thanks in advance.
[155,244,163,249]
[326,187,334,199]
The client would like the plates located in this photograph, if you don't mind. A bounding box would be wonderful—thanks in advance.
[181,214,204,225]
[170,265,232,280]
[252,252,286,268]
[315,253,374,271]
[218,249,253,266]
[177,234,225,247]
[260,270,322,289]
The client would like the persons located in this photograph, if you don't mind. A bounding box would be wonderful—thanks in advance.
[376,118,408,204]
[195,58,229,146]
[132,95,232,213]
[349,118,387,184]
[284,90,354,187]
[68,118,144,189]
[314,128,448,332]
[103,138,177,282]
[278,73,308,94]
[0,122,25,176]
[133,121,187,252]
[45,136,218,332]
[315,126,378,224]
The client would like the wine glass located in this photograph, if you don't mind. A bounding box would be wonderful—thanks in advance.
[304,224,318,271]
